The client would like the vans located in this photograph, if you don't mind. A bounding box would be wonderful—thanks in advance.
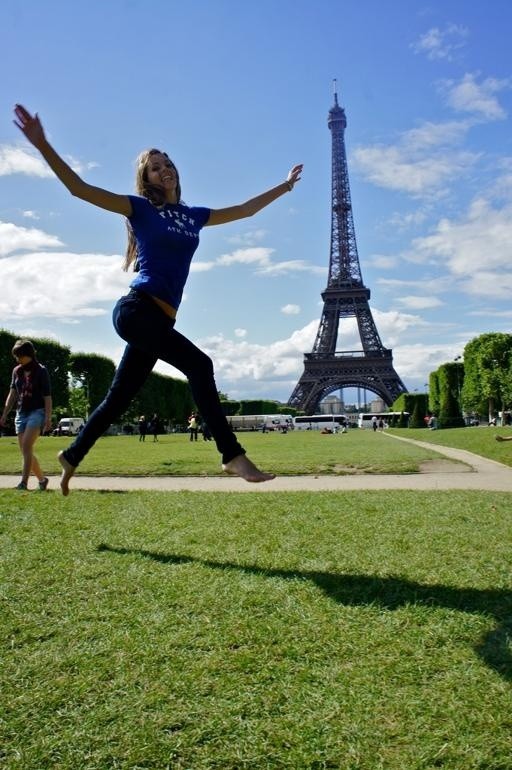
[58,417,85,437]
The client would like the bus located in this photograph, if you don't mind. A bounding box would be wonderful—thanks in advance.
[292,412,413,431]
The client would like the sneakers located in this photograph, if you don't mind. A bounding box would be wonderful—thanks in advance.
[38,478,48,490]
[15,482,27,489]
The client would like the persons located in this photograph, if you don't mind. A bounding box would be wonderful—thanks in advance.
[41,422,84,438]
[136,411,214,442]
[493,435,512,441]
[319,412,439,434]
[13,103,303,496]
[260,422,313,435]
[488,416,496,427]
[0,339,53,492]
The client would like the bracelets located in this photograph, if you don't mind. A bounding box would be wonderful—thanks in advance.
[285,180,292,192]
